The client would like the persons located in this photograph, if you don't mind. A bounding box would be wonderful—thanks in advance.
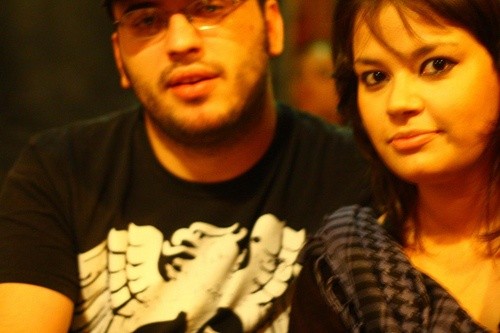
[1,0,382,333]
[290,35,348,131]
[289,1,500,333]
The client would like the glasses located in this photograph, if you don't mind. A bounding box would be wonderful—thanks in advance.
[109,0,256,37]
